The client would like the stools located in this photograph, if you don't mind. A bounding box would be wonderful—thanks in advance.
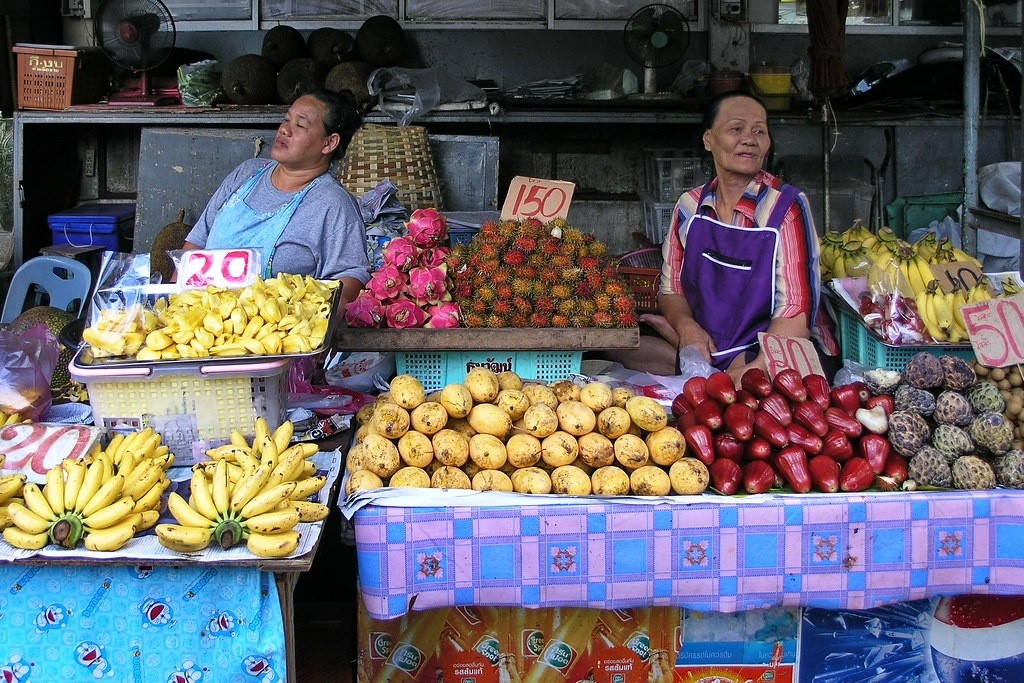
[34,244,107,311]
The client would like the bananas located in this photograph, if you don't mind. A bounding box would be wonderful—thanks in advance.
[0,411,175,552]
[156,418,330,557]
[814,219,1016,341]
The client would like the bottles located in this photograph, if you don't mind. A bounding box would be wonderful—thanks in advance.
[496,654,522,683]
[441,626,472,655]
[372,606,450,683]
[472,619,499,672]
[598,609,636,641]
[593,623,622,652]
[521,607,600,683]
[509,607,549,682]
[663,607,683,671]
[621,616,651,668]
[648,648,673,683]
[358,659,370,683]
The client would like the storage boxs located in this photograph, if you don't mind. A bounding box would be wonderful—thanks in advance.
[614,251,661,310]
[396,350,588,397]
[68,342,292,466]
[11,46,119,112]
[48,203,137,260]
[751,88,791,110]
[641,148,716,203]
[636,190,676,244]
[835,306,977,374]
[373,229,481,273]
[751,74,791,93]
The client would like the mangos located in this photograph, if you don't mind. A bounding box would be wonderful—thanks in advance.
[344,367,711,498]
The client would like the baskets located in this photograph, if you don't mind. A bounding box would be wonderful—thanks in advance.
[620,248,662,312]
[70,342,288,467]
[396,350,587,398]
[14,44,115,111]
[832,304,977,371]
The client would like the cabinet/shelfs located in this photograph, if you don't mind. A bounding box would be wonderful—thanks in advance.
[92,0,1024,36]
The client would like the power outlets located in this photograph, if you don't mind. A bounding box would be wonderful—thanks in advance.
[721,0,740,14]
[71,0,91,18]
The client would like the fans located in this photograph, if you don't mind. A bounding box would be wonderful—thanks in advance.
[93,0,180,105]
[623,3,690,102]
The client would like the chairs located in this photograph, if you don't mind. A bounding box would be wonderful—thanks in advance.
[1,256,92,319]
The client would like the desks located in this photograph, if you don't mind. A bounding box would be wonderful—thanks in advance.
[13,112,1021,272]
[0,414,358,683]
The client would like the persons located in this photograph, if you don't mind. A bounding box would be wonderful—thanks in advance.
[167,89,370,313]
[603,91,840,395]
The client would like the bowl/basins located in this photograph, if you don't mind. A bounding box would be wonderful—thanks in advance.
[326,350,395,391]
